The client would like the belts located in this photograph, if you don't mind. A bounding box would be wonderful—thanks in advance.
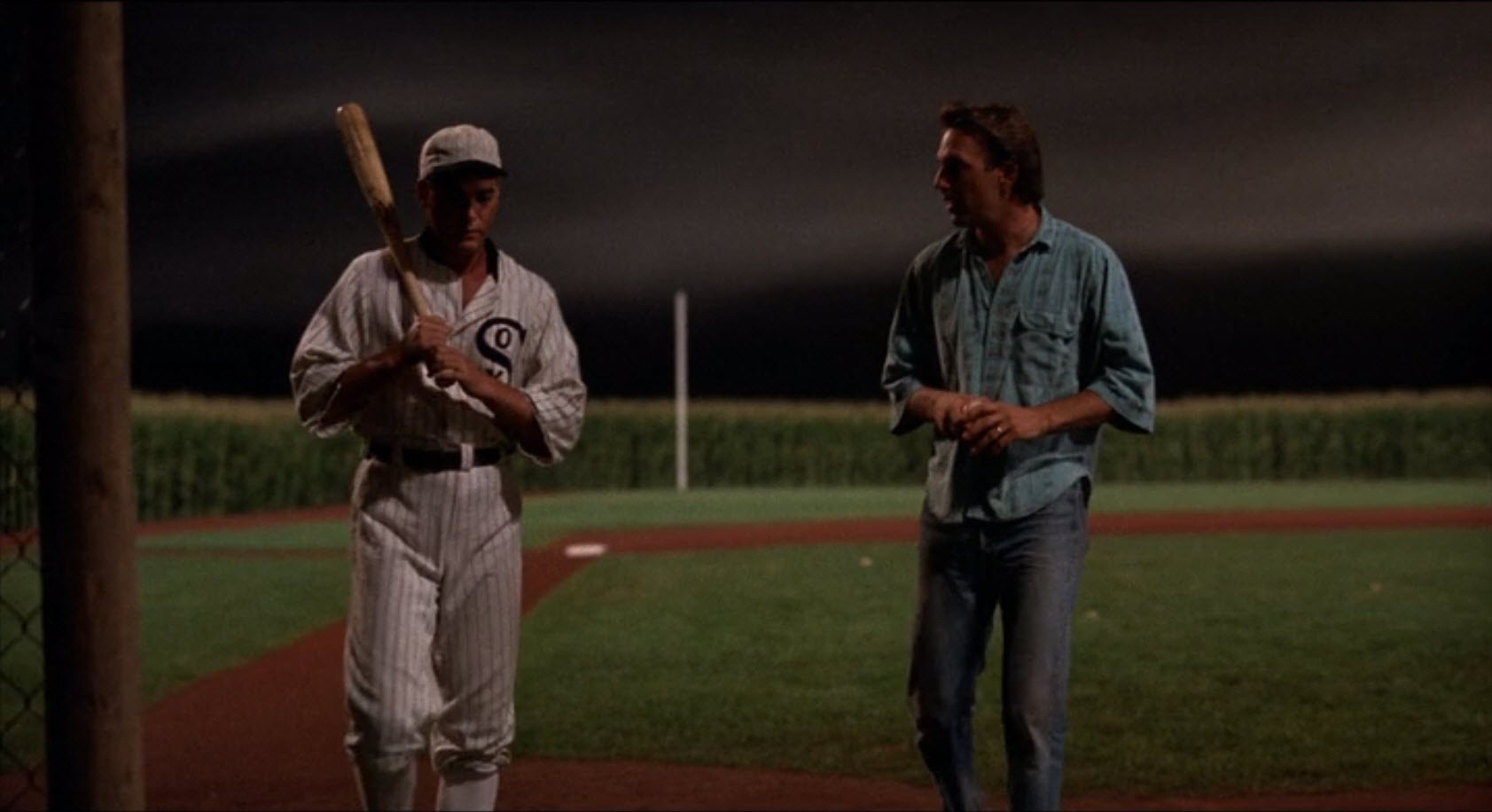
[361,435,513,472]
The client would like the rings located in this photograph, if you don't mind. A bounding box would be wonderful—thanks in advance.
[996,425,1004,433]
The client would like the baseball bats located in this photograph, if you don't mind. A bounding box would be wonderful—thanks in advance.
[335,102,457,388]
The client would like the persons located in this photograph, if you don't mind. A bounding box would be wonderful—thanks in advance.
[288,125,587,811]
[879,104,1158,812]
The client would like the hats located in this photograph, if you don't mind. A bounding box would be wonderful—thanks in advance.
[417,125,508,184]
